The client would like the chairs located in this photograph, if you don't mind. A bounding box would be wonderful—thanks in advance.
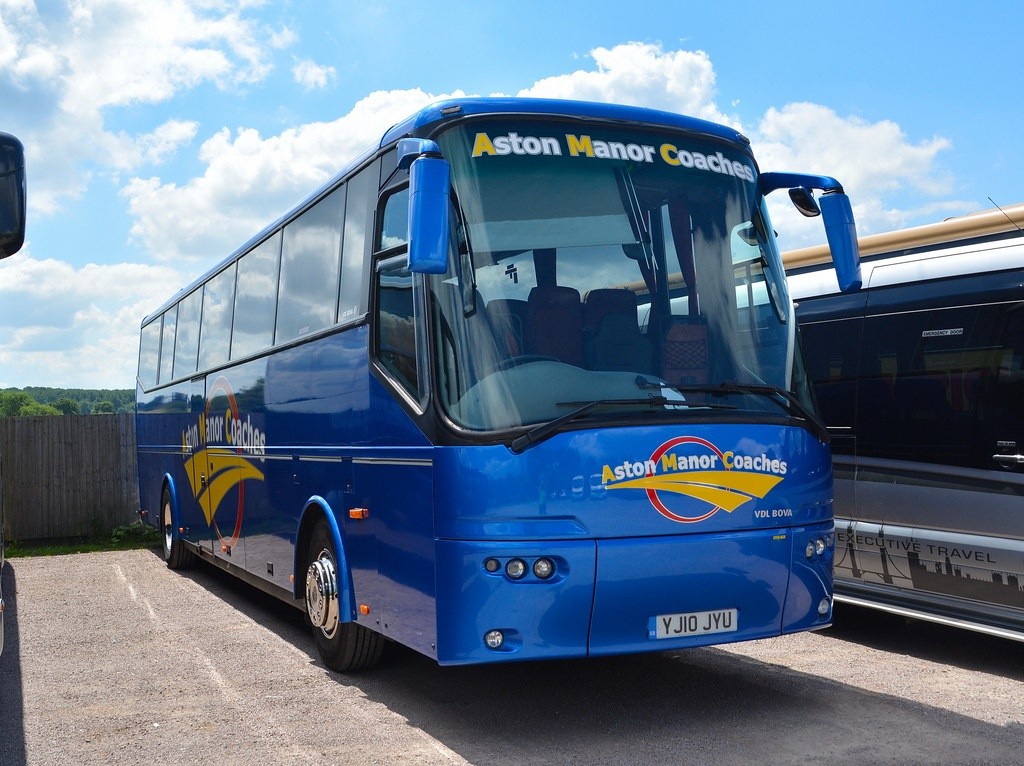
[442,281,712,405]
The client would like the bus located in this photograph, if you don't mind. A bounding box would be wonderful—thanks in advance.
[615,204,1024,647]
[133,97,865,678]
